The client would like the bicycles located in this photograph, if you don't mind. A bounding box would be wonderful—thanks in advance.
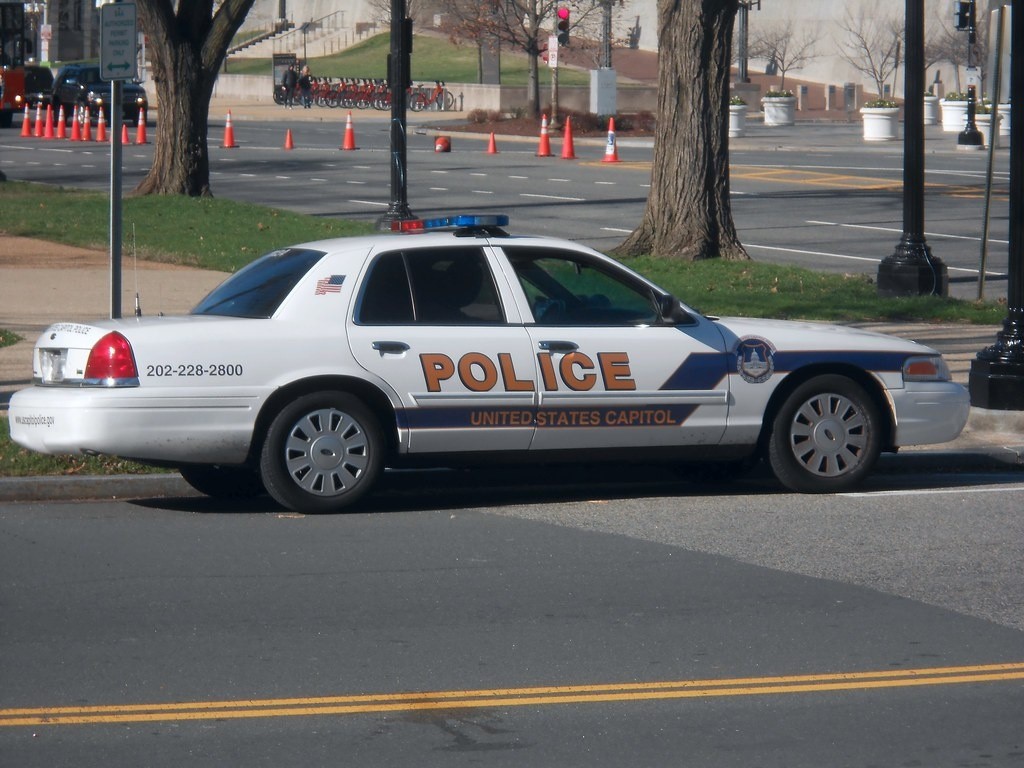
[272,77,454,112]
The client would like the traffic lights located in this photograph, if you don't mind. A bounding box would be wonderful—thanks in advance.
[556,7,569,44]
[954,1,970,28]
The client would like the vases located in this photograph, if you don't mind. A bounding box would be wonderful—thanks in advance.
[729,104,748,138]
[860,107,900,141]
[761,97,797,126]
[924,96,939,125]
[939,98,987,131]
[984,104,1011,136]
[962,114,1004,149]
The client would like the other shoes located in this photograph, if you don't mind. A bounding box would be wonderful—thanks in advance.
[289,105,292,109]
[284,104,287,108]
[308,105,311,108]
[304,106,307,108]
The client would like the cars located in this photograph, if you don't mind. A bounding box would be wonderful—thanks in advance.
[24,63,55,109]
[8,213,973,514]
[52,62,148,127]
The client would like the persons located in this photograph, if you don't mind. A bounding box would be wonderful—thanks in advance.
[298,66,313,108]
[444,261,502,321]
[281,63,297,110]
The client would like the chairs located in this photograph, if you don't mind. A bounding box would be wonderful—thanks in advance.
[429,265,477,324]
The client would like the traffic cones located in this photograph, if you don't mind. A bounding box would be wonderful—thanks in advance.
[601,117,622,162]
[218,109,240,148]
[121,124,130,143]
[559,117,579,159]
[483,132,500,154]
[534,114,556,157]
[80,106,94,141]
[283,129,295,150]
[338,110,361,150]
[132,108,152,145]
[19,103,82,140]
[95,107,109,142]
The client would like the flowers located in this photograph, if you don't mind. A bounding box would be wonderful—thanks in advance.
[945,89,992,114]
[766,89,794,97]
[924,90,932,96]
[865,98,896,108]
[729,95,747,105]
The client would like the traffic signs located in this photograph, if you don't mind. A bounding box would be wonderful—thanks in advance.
[100,2,136,80]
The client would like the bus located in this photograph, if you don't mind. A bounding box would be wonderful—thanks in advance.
[0,0,34,128]
